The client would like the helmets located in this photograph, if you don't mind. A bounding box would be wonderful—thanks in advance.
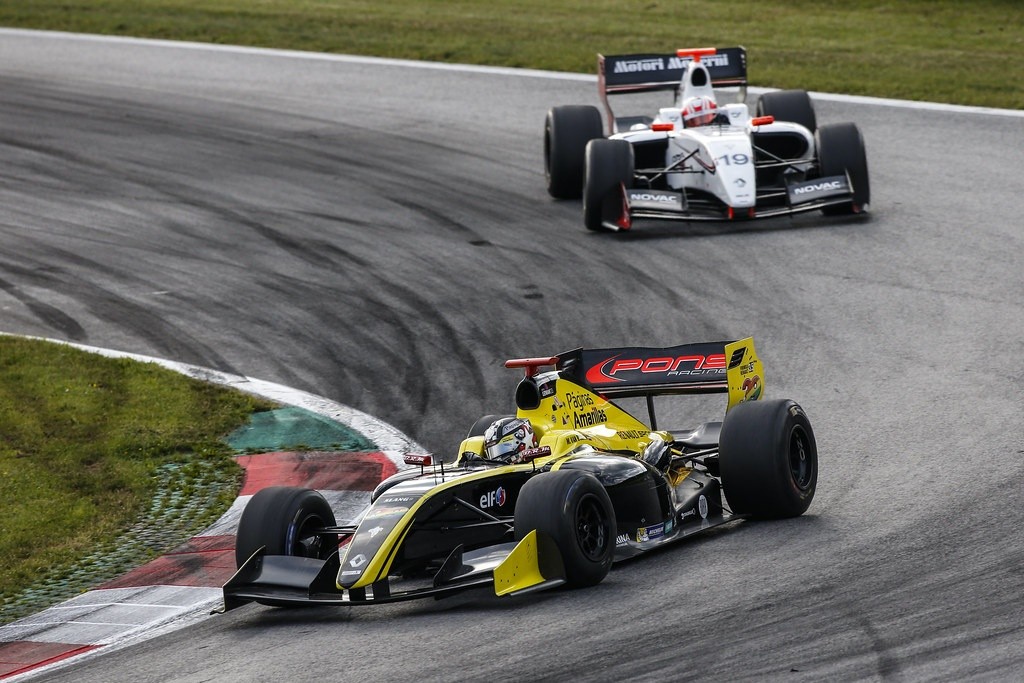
[483,417,538,463]
[682,97,719,127]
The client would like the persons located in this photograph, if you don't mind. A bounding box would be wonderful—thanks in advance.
[681,97,719,127]
[482,417,538,464]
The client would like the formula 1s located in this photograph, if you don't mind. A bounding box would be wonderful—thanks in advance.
[542,45,873,236]
[210,334,822,617]
